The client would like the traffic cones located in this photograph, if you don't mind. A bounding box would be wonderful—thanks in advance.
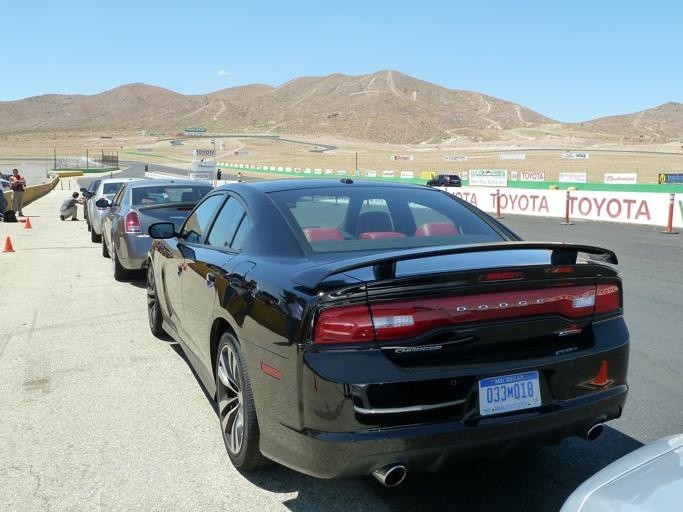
[1,236,16,252]
[23,217,33,229]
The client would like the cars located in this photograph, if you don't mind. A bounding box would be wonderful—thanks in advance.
[80,175,221,282]
[557,430,683,512]
[144,174,634,493]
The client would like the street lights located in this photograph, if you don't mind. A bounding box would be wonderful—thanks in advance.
[210,138,215,173]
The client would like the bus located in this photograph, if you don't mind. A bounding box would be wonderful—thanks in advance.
[168,140,184,145]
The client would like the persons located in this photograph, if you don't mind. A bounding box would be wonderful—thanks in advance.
[217,169,221,179]
[8,168,25,216]
[237,172,241,182]
[58,192,84,221]
[0,172,10,220]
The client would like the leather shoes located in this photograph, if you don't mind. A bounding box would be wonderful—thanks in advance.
[72,218,78,221]
[61,215,64,221]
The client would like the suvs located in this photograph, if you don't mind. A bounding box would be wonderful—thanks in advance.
[424,173,462,187]
